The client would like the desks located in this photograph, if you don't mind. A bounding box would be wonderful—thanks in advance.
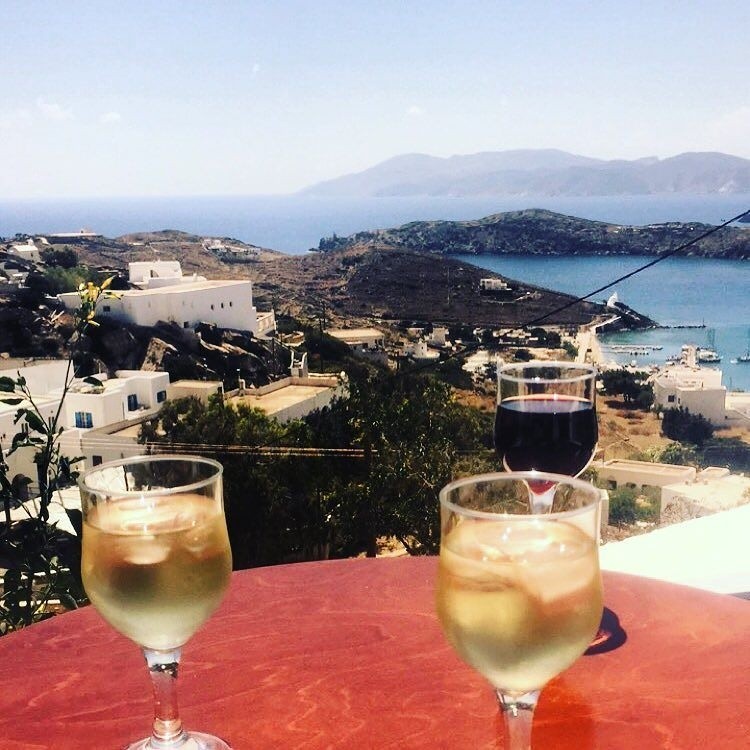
[0,558,750,750]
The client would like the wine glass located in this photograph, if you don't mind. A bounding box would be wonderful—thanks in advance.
[492,355,600,516]
[77,455,233,750]
[434,469,605,750]
